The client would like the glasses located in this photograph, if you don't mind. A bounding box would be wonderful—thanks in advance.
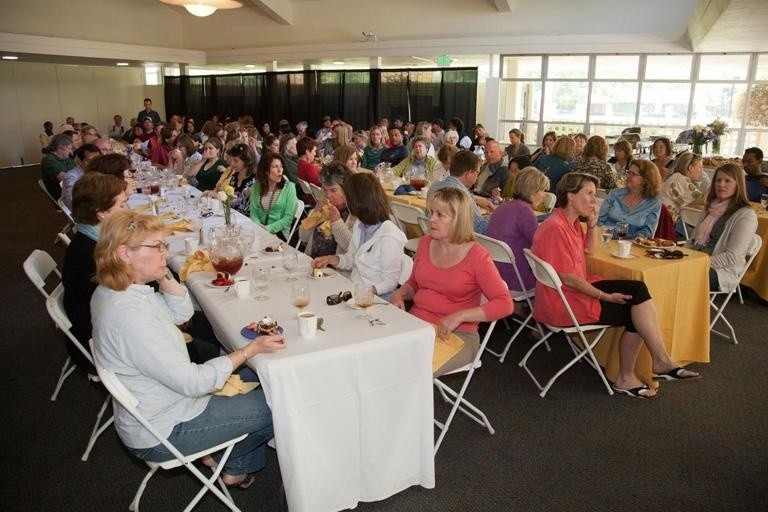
[625,168,647,178]
[135,241,173,253]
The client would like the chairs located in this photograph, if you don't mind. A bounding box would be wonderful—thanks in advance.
[23,249,96,400]
[433,279,509,456]
[82,338,250,511]
[38,125,768,397]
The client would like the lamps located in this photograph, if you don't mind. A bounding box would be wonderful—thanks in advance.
[158,0,245,21]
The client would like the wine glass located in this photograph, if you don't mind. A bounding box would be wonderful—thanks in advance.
[290,284,312,319]
[353,284,376,321]
[282,251,300,282]
[254,268,273,301]
[600,226,614,250]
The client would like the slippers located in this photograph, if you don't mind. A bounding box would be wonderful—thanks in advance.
[527,329,541,343]
[612,381,659,401]
[651,366,704,384]
[222,472,257,490]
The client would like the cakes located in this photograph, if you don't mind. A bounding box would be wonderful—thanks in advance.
[257,316,277,337]
[313,269,323,278]
[212,272,234,286]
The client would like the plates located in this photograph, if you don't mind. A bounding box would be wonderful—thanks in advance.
[611,252,639,260]
[307,274,337,279]
[203,277,233,289]
[632,237,677,249]
[240,324,282,339]
[260,245,291,254]
[345,296,380,311]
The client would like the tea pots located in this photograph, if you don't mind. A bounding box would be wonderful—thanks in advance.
[205,224,243,275]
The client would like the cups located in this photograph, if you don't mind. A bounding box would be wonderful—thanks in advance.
[233,276,251,298]
[373,162,431,200]
[184,238,199,254]
[617,240,632,256]
[616,221,630,240]
[298,311,320,339]
[132,157,220,224]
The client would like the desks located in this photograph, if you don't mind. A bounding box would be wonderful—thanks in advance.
[187,256,435,512]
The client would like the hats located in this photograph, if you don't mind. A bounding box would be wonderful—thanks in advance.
[145,117,152,122]
